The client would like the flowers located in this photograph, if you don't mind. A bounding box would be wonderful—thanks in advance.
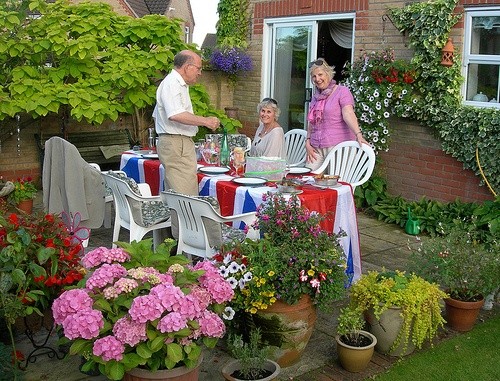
[0,168,500,381]
[210,44,254,83]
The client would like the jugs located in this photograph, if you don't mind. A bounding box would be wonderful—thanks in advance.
[229,134,251,161]
[405,207,420,235]
[205,134,224,150]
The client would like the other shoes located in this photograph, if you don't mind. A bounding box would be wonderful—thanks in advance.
[169,245,204,264]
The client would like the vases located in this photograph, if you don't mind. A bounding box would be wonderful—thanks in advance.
[221,327,281,381]
[19,199,34,214]
[123,347,205,381]
[254,290,317,368]
[441,285,485,332]
[366,297,419,356]
[333,329,378,374]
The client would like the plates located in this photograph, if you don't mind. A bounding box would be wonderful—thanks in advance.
[231,177,268,185]
[143,154,158,158]
[288,167,312,173]
[311,182,342,188]
[199,167,229,174]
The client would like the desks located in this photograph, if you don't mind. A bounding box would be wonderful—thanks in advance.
[117,146,364,293]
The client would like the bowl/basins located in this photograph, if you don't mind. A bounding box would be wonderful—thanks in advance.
[275,181,294,191]
[312,175,341,185]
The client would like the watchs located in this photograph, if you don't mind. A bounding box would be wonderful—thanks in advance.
[305,137,310,141]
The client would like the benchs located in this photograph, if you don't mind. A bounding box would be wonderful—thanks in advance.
[30,130,141,185]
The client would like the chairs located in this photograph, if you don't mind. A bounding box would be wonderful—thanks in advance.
[100,170,174,250]
[159,189,262,267]
[312,141,375,196]
[285,127,307,169]
[42,135,127,248]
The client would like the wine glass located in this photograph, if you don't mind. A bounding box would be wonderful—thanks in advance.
[240,158,246,175]
[233,157,241,177]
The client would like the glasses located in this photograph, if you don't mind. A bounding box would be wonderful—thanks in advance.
[262,98,278,108]
[309,60,327,69]
[187,62,202,72]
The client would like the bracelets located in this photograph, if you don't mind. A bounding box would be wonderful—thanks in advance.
[355,131,362,137]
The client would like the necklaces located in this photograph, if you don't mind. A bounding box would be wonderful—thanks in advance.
[255,122,273,146]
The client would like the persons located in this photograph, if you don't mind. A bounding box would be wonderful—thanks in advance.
[306,58,371,172]
[152,50,220,242]
[250,98,286,159]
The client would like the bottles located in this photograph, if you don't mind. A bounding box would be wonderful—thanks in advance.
[220,128,230,167]
